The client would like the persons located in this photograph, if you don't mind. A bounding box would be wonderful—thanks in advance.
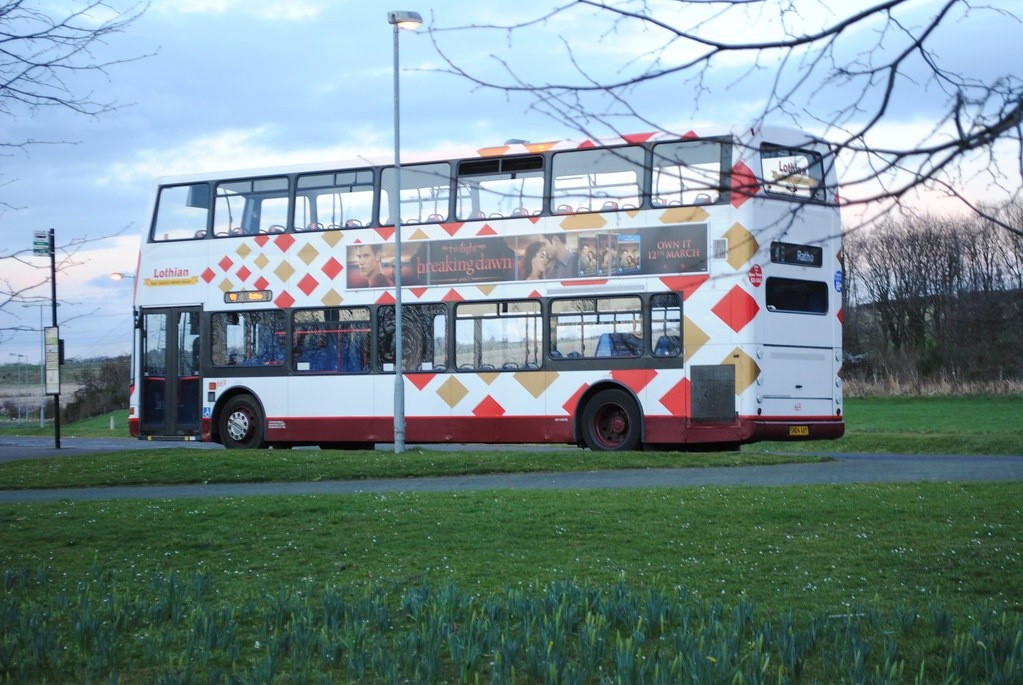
[520,233,575,280]
[356,244,393,286]
[580,243,638,273]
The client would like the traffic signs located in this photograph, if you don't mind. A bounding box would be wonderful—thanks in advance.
[32,229,50,256]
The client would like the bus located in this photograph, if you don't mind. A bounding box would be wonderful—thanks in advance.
[128,124,845,451]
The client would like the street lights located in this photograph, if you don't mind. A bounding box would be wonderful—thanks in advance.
[22,302,61,427]
[10,353,29,423]
[388,11,423,452]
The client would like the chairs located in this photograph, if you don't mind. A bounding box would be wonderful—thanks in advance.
[417,332,681,370]
[195,192,721,239]
